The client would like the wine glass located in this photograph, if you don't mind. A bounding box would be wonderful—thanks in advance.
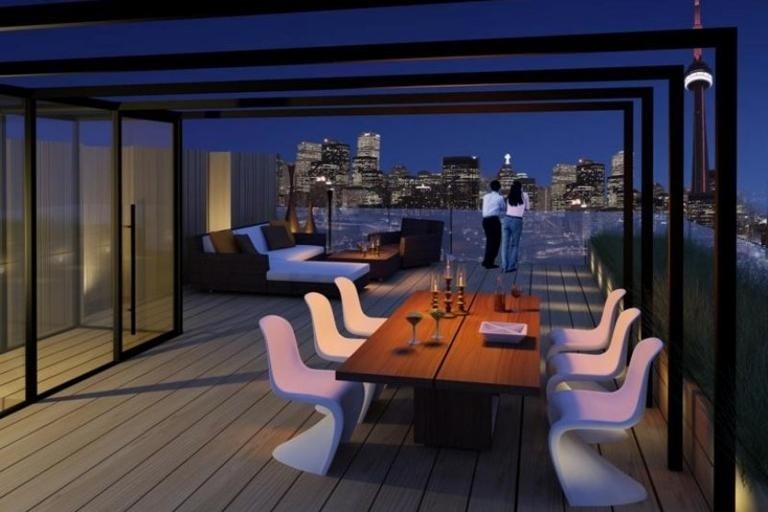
[429,304,446,339]
[406,306,424,345]
[511,279,520,312]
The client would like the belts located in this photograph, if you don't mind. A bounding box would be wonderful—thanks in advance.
[506,214,522,220]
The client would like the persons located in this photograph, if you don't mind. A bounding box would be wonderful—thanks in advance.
[477,180,506,269]
[501,180,530,273]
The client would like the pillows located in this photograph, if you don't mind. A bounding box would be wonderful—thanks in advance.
[233,234,260,256]
[271,218,296,244]
[208,228,236,254]
[259,225,294,252]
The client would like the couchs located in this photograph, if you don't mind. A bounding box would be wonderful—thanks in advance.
[184,217,371,301]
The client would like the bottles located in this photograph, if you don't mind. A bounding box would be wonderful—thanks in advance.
[494,273,505,313]
[360,237,381,258]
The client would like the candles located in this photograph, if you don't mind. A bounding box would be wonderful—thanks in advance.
[459,272,463,286]
[433,280,438,291]
[446,260,450,278]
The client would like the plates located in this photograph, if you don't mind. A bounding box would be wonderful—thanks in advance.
[478,321,529,345]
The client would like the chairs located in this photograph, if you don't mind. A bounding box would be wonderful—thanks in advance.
[301,291,385,426]
[365,216,445,271]
[331,275,391,392]
[541,305,643,444]
[545,334,664,505]
[542,285,629,394]
[257,313,366,478]
